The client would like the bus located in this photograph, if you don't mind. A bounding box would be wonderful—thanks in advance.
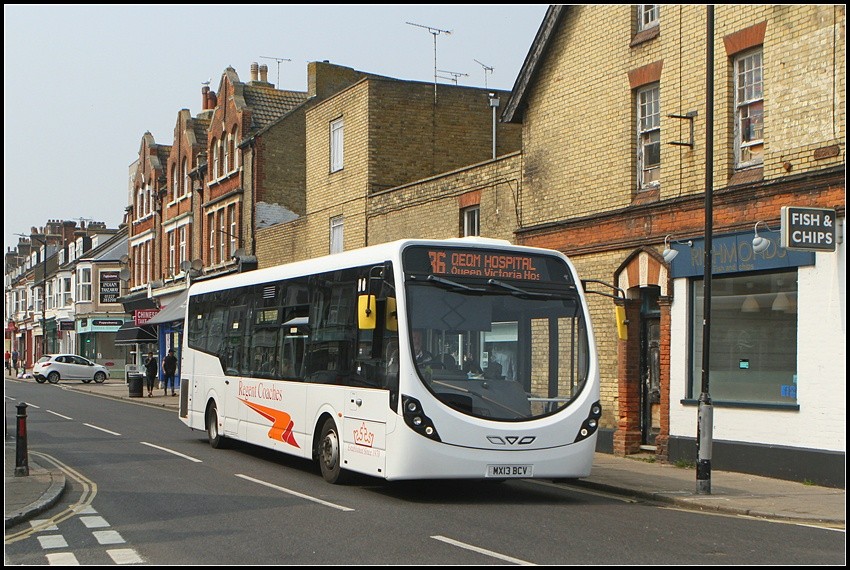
[179,237,629,492]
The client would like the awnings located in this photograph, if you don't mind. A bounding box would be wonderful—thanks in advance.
[114,322,158,344]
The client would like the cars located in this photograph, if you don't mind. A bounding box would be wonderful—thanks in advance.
[32,353,110,385]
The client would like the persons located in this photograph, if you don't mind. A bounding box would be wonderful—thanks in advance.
[12,349,18,369]
[387,330,434,382]
[5,351,11,368]
[162,348,177,397]
[144,352,157,397]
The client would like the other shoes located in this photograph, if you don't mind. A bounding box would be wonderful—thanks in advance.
[164,393,167,395]
[151,393,152,396]
[148,393,150,396]
[172,393,177,397]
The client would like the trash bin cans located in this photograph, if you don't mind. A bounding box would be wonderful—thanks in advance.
[128,373,143,397]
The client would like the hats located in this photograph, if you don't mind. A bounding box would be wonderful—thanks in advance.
[14,349,16,351]
[169,349,176,352]
